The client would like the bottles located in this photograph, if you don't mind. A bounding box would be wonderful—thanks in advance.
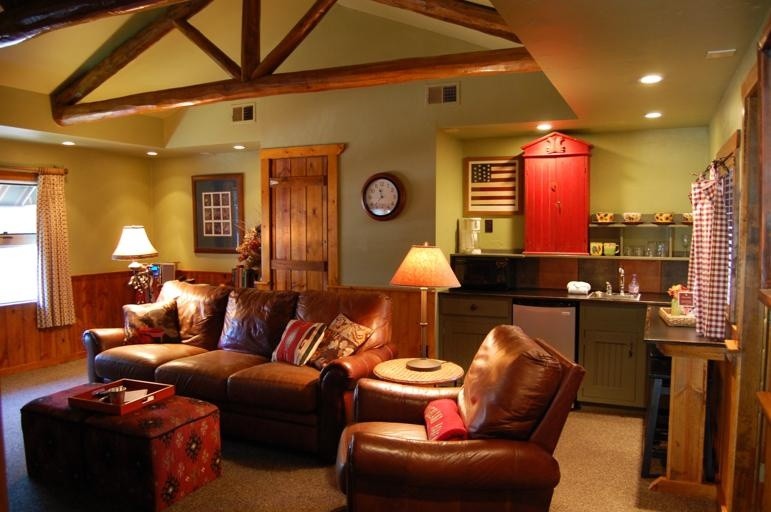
[644,240,667,257]
[627,272,640,297]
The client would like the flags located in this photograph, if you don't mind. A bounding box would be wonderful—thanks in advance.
[469,162,516,207]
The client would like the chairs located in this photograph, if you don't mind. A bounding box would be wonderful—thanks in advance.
[336,326,587,511]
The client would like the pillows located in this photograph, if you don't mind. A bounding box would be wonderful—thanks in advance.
[307,314,375,371]
[272,319,328,366]
[123,302,181,344]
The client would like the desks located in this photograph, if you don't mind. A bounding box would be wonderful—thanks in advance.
[20,383,222,511]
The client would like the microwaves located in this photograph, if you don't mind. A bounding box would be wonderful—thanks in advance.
[450,255,515,292]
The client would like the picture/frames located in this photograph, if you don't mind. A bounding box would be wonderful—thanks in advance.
[464,156,524,218]
[189,172,246,252]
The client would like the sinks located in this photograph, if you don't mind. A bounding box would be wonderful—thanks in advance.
[585,290,642,302]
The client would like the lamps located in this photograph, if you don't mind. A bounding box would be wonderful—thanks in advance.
[111,226,159,305]
[391,245,461,370]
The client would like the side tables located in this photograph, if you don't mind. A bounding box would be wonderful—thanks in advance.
[373,357,465,387]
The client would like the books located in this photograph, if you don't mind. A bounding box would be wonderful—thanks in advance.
[231,266,255,289]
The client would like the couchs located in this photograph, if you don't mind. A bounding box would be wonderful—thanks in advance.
[83,279,398,462]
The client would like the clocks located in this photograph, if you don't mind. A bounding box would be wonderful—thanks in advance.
[361,172,406,221]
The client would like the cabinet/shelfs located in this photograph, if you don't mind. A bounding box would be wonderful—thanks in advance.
[437,213,694,413]
[520,132,592,256]
[644,304,726,504]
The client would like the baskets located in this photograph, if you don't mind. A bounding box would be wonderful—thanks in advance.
[658,307,696,327]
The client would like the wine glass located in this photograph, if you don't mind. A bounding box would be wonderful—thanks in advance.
[680,233,691,257]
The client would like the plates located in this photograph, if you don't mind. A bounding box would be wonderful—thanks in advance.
[622,222,643,225]
[652,221,676,225]
[681,222,695,225]
[593,221,617,224]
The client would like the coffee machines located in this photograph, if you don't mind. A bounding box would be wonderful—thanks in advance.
[457,216,481,255]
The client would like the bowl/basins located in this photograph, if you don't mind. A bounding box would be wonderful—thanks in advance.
[595,211,614,223]
[681,212,694,221]
[655,212,674,223]
[622,212,641,222]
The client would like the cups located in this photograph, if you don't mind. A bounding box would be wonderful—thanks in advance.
[589,241,604,256]
[624,245,643,256]
[107,387,126,405]
[604,242,619,257]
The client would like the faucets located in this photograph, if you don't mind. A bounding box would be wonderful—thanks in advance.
[605,281,612,293]
[617,266,625,295]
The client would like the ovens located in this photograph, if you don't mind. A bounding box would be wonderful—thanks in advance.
[513,298,579,411]
